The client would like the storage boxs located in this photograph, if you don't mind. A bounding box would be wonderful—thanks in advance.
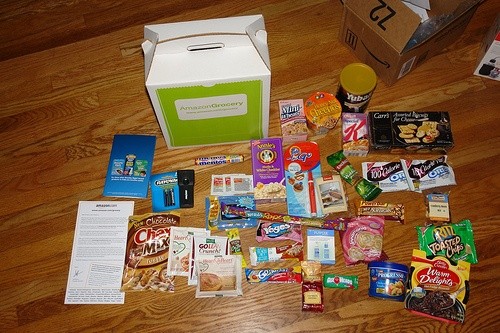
[339,1,483,90]
[140,14,273,149]
[0,1,500,333]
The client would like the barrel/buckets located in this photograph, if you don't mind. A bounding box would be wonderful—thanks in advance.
[367,261,409,302]
